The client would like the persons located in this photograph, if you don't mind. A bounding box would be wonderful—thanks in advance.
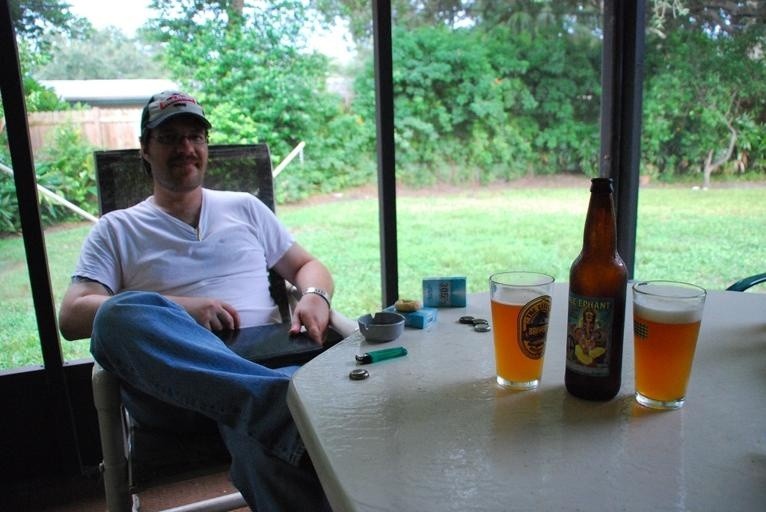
[56,88,337,512]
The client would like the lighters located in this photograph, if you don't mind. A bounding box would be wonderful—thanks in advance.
[355,346,408,365]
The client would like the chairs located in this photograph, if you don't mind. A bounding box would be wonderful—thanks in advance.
[92,143,357,510]
[725,273,766,292]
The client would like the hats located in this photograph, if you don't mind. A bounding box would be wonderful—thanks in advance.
[141,91,212,135]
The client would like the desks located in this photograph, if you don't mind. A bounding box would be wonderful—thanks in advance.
[286,283,766,512]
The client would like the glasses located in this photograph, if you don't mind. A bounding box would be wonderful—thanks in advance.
[148,134,208,144]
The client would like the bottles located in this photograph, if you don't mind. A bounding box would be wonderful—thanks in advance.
[563,178,628,401]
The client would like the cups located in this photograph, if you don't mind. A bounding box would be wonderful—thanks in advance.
[632,280,707,410]
[488,272,555,391]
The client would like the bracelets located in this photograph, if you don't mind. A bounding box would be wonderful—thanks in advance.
[303,286,332,309]
